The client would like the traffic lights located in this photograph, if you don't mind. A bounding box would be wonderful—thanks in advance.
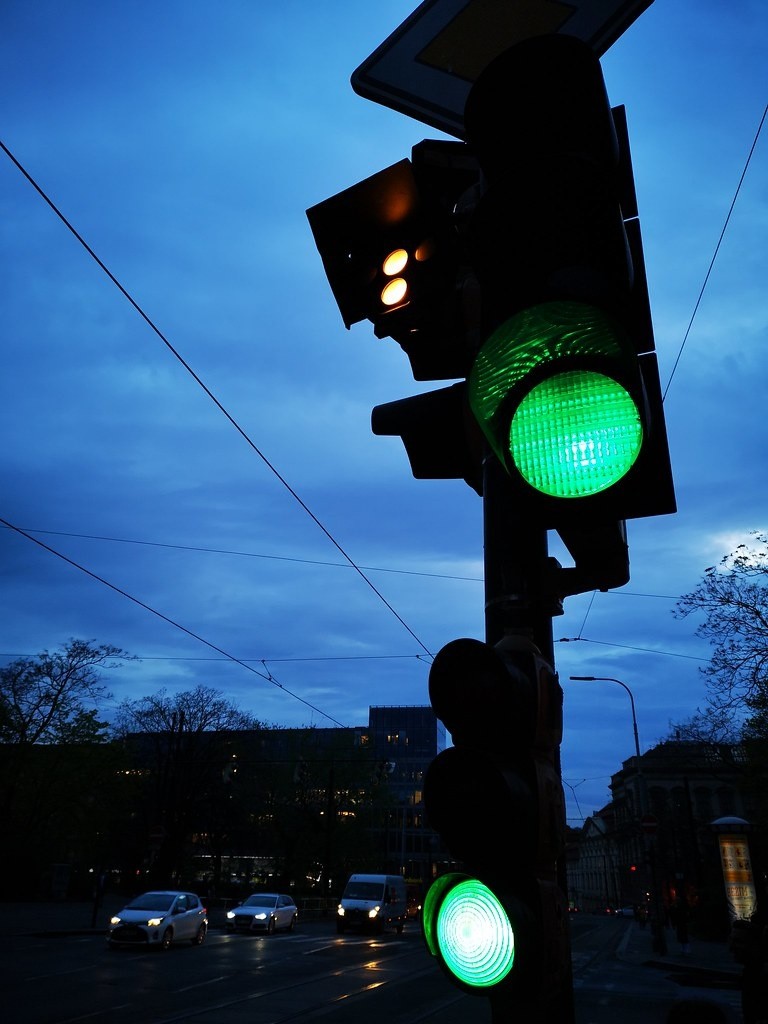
[463,30,677,535]
[418,629,566,997]
[365,233,418,336]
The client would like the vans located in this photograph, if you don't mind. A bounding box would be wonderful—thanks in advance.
[337,873,408,936]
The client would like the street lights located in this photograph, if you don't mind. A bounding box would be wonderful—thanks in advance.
[569,675,667,953]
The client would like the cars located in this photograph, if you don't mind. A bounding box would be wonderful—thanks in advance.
[106,888,209,951]
[615,905,635,918]
[224,892,300,933]
